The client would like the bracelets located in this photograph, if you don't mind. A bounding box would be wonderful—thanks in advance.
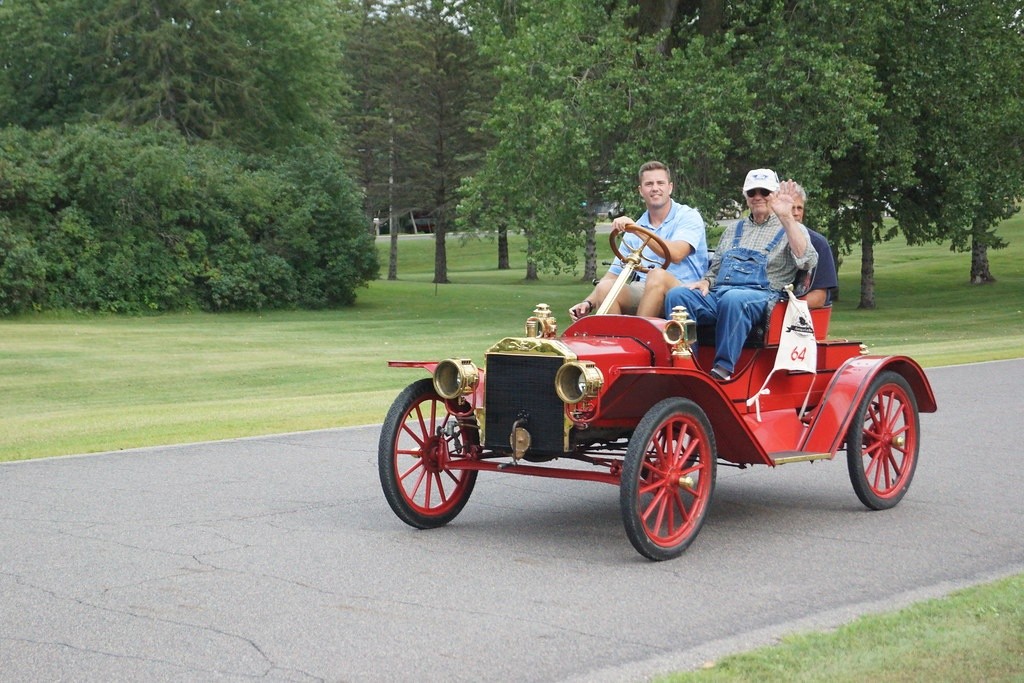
[583,300,593,313]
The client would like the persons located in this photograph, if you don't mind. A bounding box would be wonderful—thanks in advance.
[568,160,710,324]
[777,181,838,310]
[662,169,819,383]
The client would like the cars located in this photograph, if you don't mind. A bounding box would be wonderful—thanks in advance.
[374,224,939,562]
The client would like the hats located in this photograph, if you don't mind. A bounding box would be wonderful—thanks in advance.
[743,168,780,199]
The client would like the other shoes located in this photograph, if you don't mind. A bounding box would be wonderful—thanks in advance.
[709,366,730,381]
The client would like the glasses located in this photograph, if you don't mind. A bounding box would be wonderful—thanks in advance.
[746,188,772,197]
